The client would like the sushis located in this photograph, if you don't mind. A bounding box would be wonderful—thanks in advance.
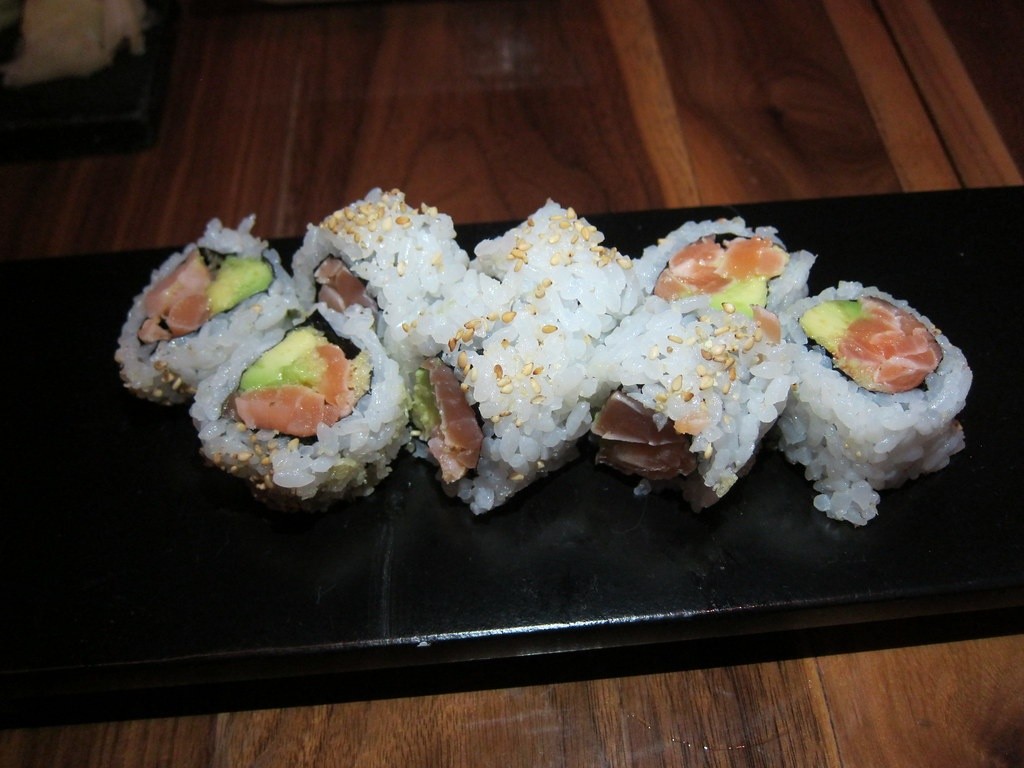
[113,185,971,526]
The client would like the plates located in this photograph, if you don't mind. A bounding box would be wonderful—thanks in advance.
[0,1,178,150]
[0,179,1024,695]
